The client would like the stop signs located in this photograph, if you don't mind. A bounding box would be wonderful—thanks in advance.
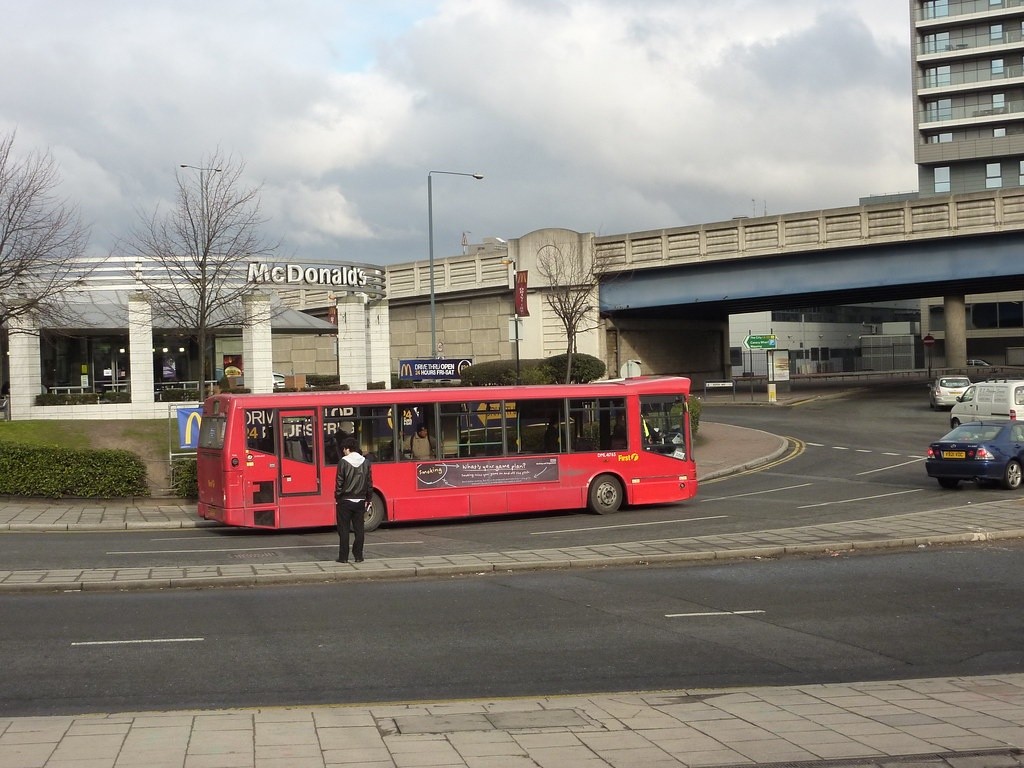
[922,335,935,347]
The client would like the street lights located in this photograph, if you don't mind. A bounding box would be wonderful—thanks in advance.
[501,258,520,383]
[606,328,620,378]
[180,164,223,254]
[627,360,641,376]
[428,170,483,381]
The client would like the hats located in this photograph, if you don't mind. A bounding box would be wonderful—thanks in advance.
[417,423,428,433]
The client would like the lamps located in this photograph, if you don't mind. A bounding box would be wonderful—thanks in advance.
[819,334,823,337]
[788,334,792,338]
[847,334,851,337]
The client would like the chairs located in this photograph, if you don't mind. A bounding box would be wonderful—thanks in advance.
[576,437,594,450]
[1010,426,1024,441]
[985,432,996,439]
[610,435,627,448]
[286,436,342,464]
[959,432,971,437]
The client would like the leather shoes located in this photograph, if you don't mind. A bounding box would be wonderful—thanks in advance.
[355,558,364,562]
[336,559,348,563]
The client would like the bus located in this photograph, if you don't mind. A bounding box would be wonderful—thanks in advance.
[197,376,698,532]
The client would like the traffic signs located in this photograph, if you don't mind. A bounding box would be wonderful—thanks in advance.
[742,334,776,349]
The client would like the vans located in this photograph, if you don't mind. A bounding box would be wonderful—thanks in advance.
[950,376,1024,429]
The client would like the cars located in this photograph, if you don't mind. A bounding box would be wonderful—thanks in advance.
[966,360,991,366]
[927,376,971,409]
[271,372,287,388]
[922,420,1024,488]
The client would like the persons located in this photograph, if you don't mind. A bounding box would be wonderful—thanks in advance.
[642,413,662,442]
[410,423,437,460]
[334,438,373,563]
[334,421,375,462]
[289,428,311,456]
[544,419,559,450]
